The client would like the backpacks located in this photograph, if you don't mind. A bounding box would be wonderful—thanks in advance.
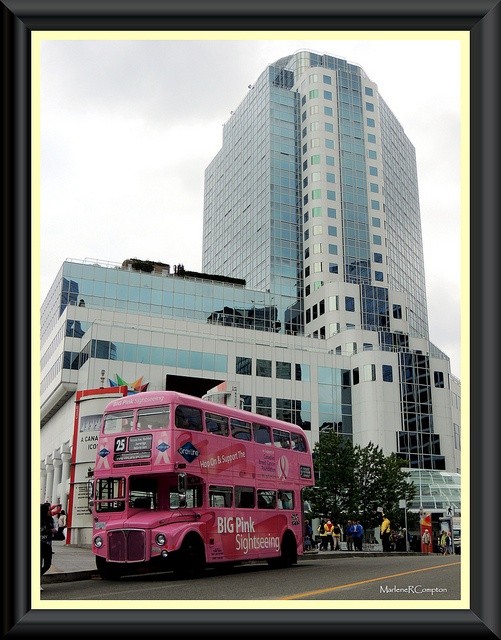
[380,530,386,539]
[334,526,341,534]
[320,524,325,534]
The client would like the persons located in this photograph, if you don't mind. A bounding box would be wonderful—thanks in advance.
[441,531,447,556]
[380,513,391,551]
[445,532,453,554]
[325,518,334,551]
[304,520,315,550]
[346,521,364,551]
[40,503,58,591]
[422,528,431,552]
[389,528,412,551]
[437,530,444,554]
[331,522,343,550]
[371,537,379,544]
[57,509,67,540]
[317,520,329,551]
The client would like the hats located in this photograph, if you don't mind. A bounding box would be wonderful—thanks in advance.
[425,529,428,531]
[402,528,406,530]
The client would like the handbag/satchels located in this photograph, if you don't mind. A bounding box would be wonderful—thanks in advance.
[305,540,311,545]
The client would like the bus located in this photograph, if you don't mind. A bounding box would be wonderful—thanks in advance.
[86,391,316,579]
